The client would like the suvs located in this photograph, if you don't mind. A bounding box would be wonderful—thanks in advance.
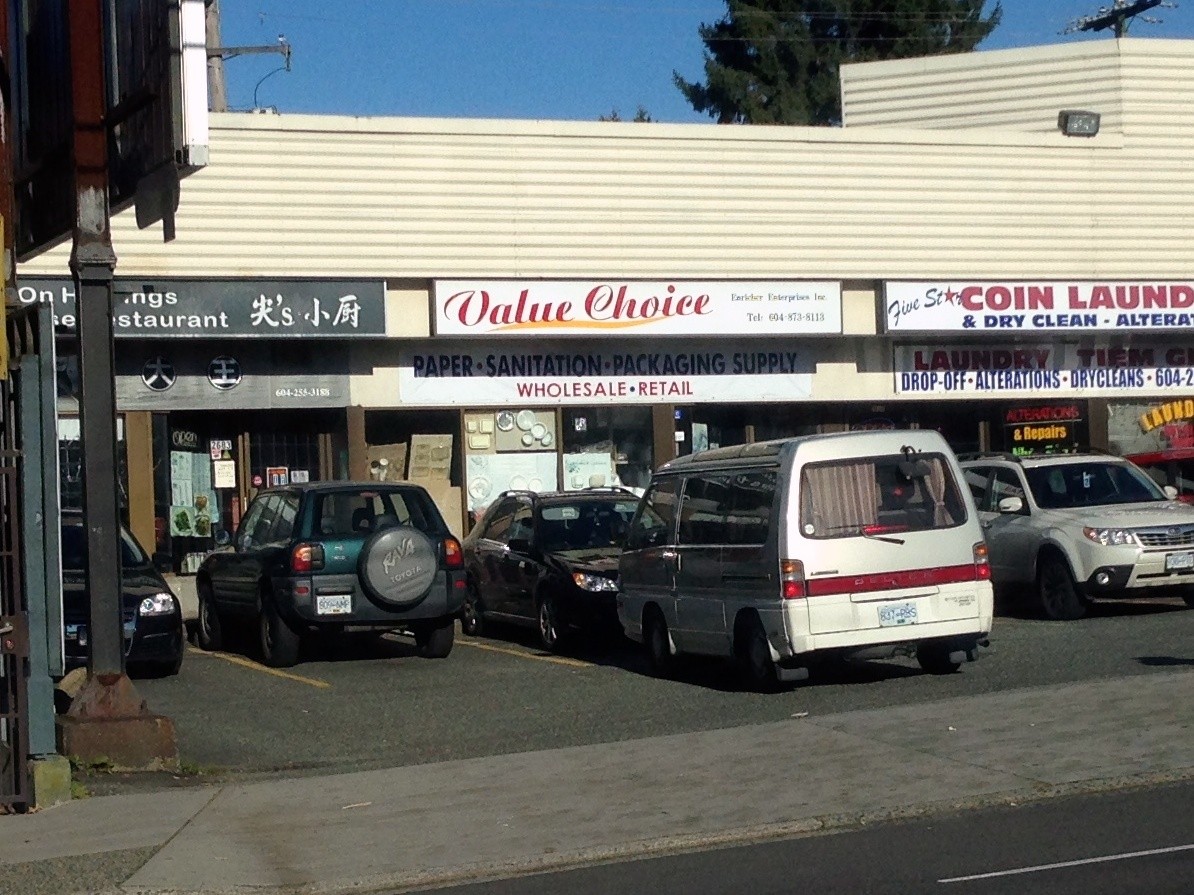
[951,446,1194,622]
[195,482,468,668]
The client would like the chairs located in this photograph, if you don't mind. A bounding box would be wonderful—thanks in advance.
[1076,472,1113,502]
[1038,474,1059,506]
[874,480,934,525]
[373,510,404,530]
[352,505,376,533]
[322,513,344,534]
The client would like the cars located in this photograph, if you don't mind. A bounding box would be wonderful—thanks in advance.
[1122,446,1194,507]
[460,485,671,653]
[59,507,186,679]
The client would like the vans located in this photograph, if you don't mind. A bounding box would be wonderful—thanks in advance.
[613,428,995,696]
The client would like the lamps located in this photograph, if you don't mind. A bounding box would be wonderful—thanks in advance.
[1055,107,1102,138]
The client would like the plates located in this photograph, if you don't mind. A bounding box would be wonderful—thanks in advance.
[467,474,545,500]
[496,409,554,446]
